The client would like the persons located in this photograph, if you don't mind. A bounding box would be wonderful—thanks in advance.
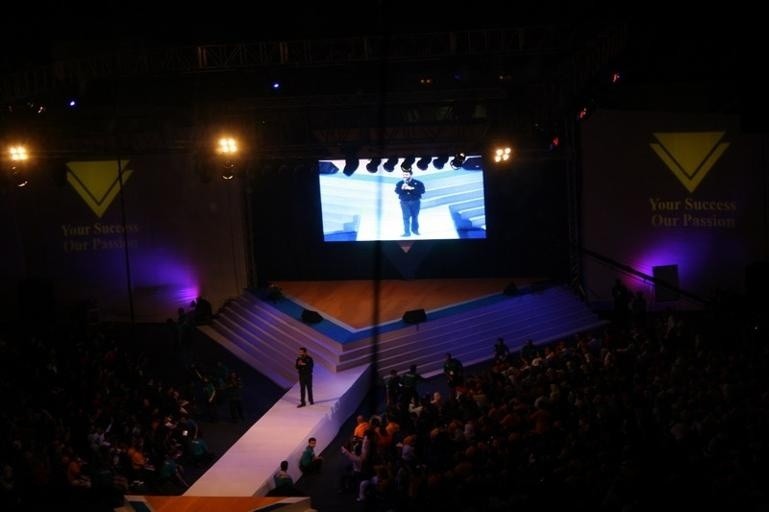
[338,278,769,512]
[273,460,297,497]
[298,438,326,480]
[294,347,313,408]
[0,292,245,511]
[394,170,425,238]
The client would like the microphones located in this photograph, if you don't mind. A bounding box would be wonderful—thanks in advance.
[404,178,407,184]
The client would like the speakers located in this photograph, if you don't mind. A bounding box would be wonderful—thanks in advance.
[301,310,323,323]
[504,281,519,296]
[402,308,426,324]
[653,264,680,302]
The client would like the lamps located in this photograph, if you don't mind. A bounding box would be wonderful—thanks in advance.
[309,159,320,177]
[221,158,235,181]
[10,165,29,187]
[343,145,466,177]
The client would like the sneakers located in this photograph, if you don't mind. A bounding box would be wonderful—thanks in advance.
[296,401,313,408]
[402,232,421,237]
